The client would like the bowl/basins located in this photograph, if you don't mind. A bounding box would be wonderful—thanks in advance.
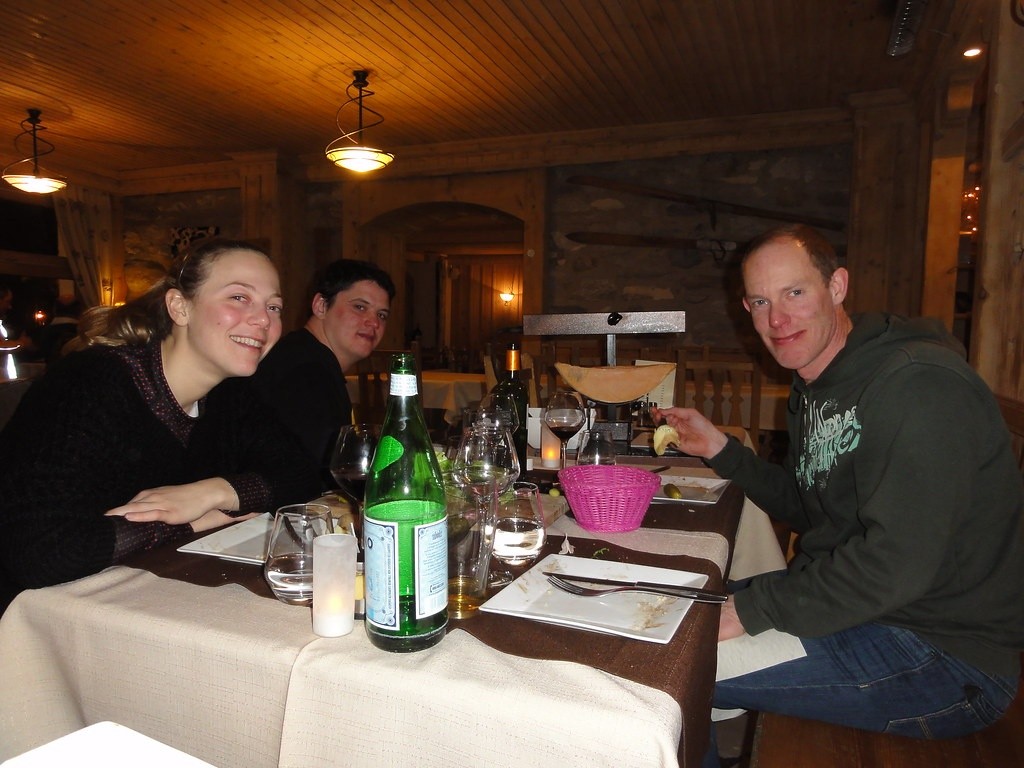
[528,408,596,450]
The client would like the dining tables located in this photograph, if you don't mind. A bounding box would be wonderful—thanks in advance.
[1,435,759,768]
[341,369,491,429]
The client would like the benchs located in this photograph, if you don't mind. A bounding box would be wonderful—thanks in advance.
[755,392,1024,768]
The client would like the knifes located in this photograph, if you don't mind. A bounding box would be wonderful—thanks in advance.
[541,571,728,601]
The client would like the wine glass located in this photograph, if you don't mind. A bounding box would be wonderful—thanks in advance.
[475,394,522,467]
[544,389,587,469]
[453,423,520,585]
[330,423,381,556]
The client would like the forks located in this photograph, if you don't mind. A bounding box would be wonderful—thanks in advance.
[547,575,726,604]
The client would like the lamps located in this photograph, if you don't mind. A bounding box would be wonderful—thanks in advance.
[325,69,396,173]
[1,108,67,194]
[500,294,515,306]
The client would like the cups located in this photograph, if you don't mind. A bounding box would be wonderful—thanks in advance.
[311,534,357,638]
[439,469,497,621]
[576,429,616,466]
[542,426,560,468]
[493,482,549,569]
[264,503,334,606]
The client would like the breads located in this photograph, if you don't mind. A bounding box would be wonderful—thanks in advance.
[336,513,359,532]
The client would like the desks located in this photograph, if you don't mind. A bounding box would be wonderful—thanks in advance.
[672,380,792,440]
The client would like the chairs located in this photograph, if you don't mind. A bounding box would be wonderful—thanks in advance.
[570,343,765,450]
[358,342,492,441]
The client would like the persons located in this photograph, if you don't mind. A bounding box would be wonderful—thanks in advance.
[0,236,321,617]
[649,225,1024,768]
[221,259,396,512]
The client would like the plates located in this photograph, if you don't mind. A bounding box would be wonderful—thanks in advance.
[176,513,273,564]
[651,474,732,504]
[476,552,709,645]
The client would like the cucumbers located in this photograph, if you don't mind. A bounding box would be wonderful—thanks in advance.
[663,483,682,498]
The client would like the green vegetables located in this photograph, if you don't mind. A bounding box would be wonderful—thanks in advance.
[413,451,515,504]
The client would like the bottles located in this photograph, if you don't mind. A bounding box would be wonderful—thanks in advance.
[637,402,657,427]
[363,353,449,653]
[488,341,528,481]
